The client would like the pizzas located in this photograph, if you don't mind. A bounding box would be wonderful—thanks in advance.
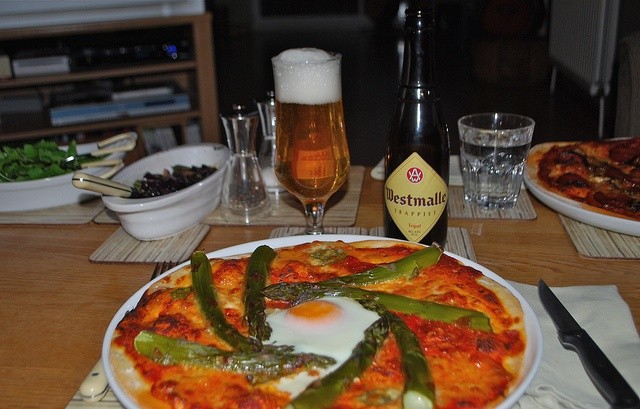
[527,138,640,221]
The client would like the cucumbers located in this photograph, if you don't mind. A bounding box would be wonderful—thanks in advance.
[293,243,443,306]
[260,282,491,332]
[133,329,336,377]
[191,250,294,355]
[286,315,387,409]
[244,244,277,339]
[359,299,436,409]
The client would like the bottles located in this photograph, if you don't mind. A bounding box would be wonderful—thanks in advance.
[255,97,289,194]
[383,6,451,251]
[219,100,270,215]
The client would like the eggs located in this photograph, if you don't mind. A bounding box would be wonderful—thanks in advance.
[260,296,381,397]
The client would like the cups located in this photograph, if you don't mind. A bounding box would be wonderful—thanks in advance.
[457,111,535,211]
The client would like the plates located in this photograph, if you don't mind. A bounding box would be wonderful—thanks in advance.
[0,131,138,214]
[524,136,640,236]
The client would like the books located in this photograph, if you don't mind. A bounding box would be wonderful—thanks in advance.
[140,122,202,156]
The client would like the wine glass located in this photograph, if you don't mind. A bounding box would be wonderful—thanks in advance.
[273,50,351,235]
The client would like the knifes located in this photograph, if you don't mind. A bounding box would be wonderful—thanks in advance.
[537,277,640,409]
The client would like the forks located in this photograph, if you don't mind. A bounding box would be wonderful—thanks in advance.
[78,260,180,401]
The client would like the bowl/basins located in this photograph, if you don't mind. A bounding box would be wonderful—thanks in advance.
[100,142,229,242]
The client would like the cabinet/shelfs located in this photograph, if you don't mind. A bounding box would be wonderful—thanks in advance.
[1,0,219,163]
